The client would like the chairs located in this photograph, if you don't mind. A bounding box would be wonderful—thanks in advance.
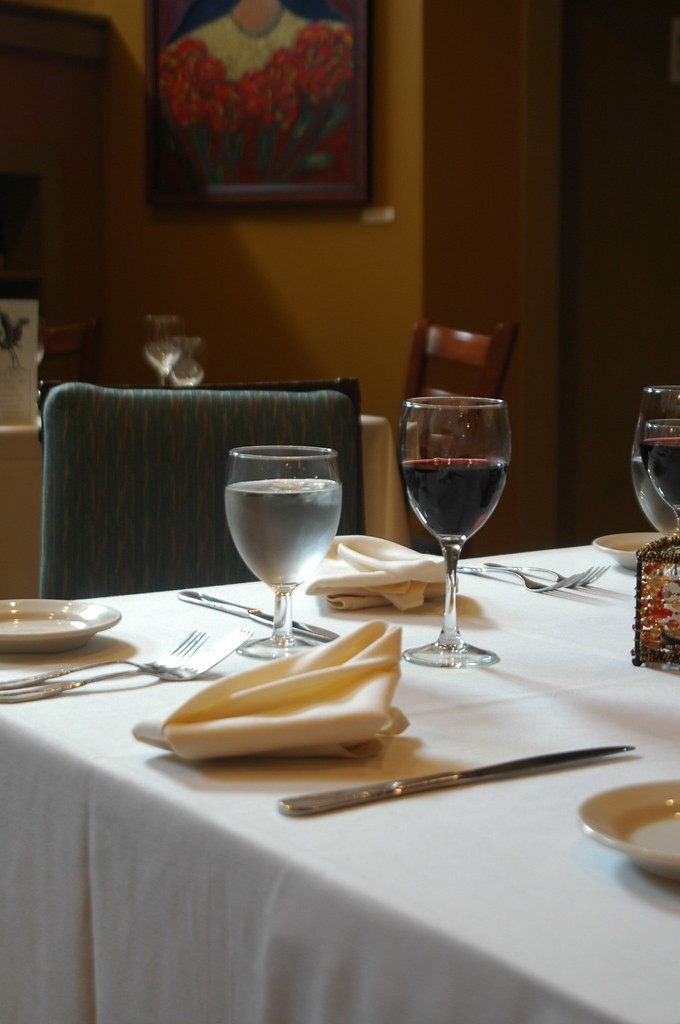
[42,319,102,384]
[404,317,521,457]
[35,376,367,600]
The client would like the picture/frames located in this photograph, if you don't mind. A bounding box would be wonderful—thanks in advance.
[142,1,374,214]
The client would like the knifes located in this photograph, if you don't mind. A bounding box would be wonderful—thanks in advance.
[277,745,637,815]
[176,590,340,642]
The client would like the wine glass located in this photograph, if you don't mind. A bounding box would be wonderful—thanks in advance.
[641,416,680,526]
[141,314,184,387]
[401,394,512,669]
[224,446,342,661]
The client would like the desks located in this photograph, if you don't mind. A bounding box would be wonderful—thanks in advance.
[0,545,680,1024]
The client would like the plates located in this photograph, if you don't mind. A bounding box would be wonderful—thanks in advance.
[575,778,680,881]
[0,598,122,654]
[592,531,668,571]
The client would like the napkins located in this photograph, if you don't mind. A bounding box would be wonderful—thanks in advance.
[304,535,446,613]
[129,621,410,757]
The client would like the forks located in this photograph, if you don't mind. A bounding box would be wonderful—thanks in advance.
[483,562,611,588]
[0,627,253,703]
[0,629,210,690]
[456,565,585,592]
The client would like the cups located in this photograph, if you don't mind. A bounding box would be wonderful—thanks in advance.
[629,416,676,535]
[170,336,206,387]
